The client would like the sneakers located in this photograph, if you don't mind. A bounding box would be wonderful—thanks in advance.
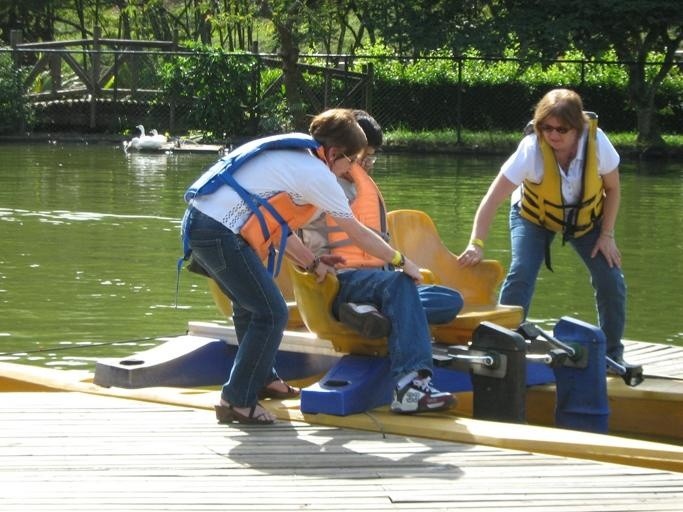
[615,355,642,371]
[390,366,460,415]
[337,300,392,340]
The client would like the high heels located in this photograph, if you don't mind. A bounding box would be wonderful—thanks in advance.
[257,375,300,401]
[214,399,275,425]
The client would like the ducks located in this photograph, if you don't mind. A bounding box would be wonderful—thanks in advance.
[122,125,168,154]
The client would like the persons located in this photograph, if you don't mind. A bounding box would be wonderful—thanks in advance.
[176,106,423,430]
[454,87,635,377]
[299,108,464,415]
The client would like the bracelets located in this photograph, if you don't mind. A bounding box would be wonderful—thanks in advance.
[600,227,616,238]
[470,237,485,250]
[391,250,401,267]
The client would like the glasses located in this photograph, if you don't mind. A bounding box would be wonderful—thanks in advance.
[537,122,575,135]
[361,153,377,170]
[340,151,358,166]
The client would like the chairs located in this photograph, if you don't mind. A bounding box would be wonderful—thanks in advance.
[206,250,305,330]
[287,259,390,357]
[386,209,524,344]
